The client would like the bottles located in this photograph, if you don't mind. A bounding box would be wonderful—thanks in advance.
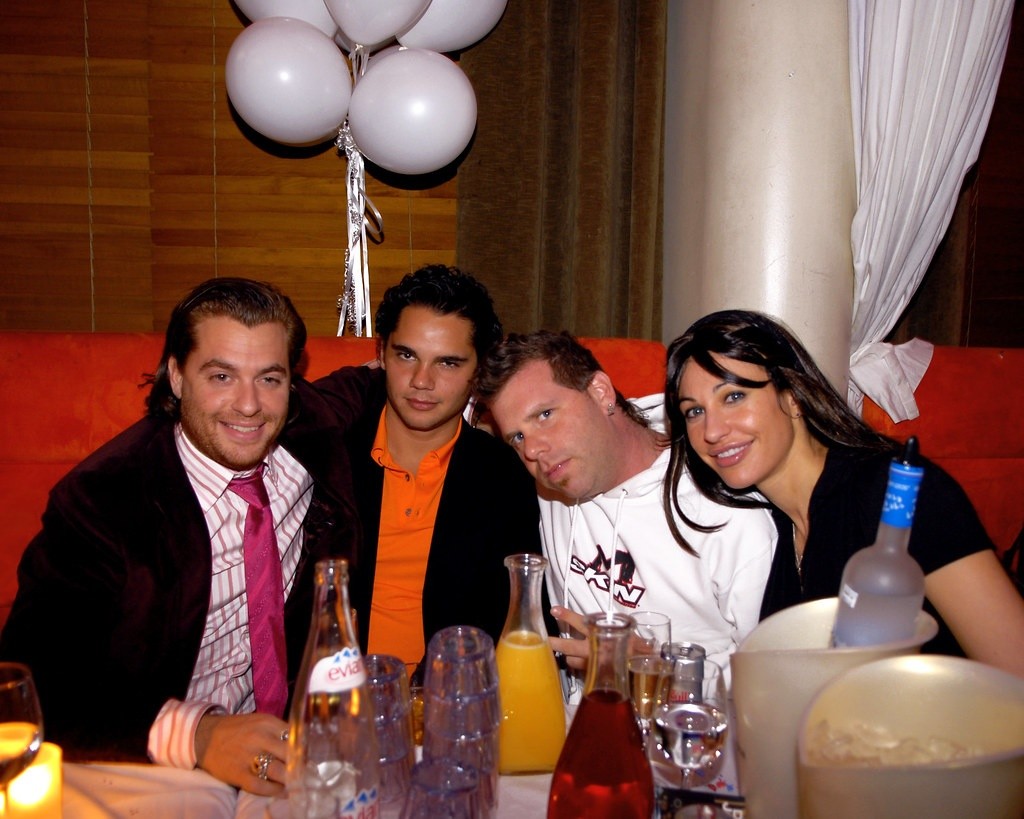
[286,560,380,819]
[829,435,926,649]
[545,613,655,819]
[493,555,566,774]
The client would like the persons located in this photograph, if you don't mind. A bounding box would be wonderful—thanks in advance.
[0,279,317,800]
[280,264,572,694]
[662,310,1024,680]
[477,329,780,700]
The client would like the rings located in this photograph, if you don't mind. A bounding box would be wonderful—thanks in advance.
[252,752,273,780]
[281,729,289,741]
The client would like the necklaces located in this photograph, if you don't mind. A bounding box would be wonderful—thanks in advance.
[792,524,803,571]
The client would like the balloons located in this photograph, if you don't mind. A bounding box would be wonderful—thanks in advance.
[323,0,433,45]
[349,46,478,174]
[225,16,353,144]
[396,0,507,53]
[335,28,394,55]
[235,0,339,41]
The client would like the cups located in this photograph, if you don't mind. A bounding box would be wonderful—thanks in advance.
[360,627,498,818]
[405,663,428,745]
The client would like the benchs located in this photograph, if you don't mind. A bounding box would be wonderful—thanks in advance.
[862,348,1024,569]
[0,329,667,626]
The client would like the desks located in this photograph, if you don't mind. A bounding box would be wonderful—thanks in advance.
[63,705,583,819]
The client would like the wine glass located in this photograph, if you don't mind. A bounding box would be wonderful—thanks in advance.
[631,610,731,819]
[0,663,43,819]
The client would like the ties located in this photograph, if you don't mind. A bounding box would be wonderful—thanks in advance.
[226,462,289,721]
